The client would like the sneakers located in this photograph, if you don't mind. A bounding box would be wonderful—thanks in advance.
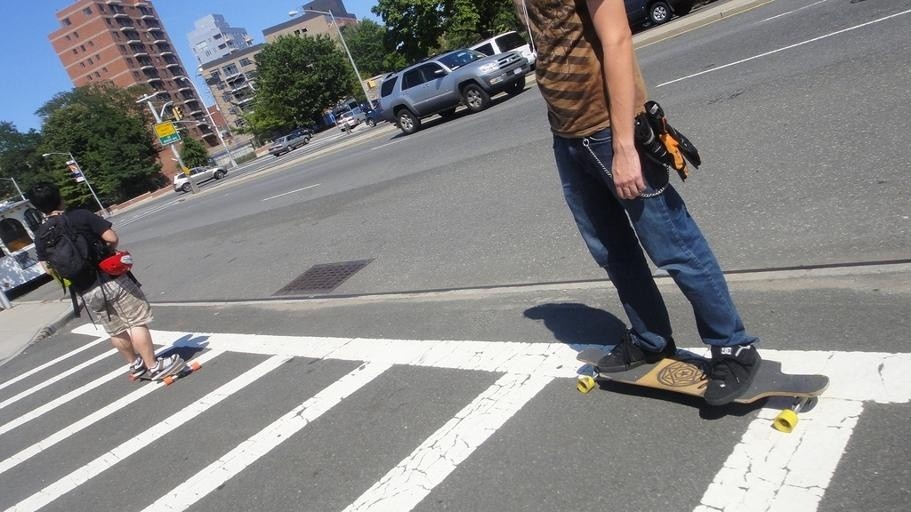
[704,343,761,407]
[597,328,676,372]
[130,356,146,378]
[147,353,180,381]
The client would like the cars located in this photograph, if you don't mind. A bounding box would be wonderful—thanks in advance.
[365,106,386,129]
[625,1,696,31]
[290,127,313,138]
[268,133,311,159]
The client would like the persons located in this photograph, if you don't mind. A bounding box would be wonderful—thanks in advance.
[525,0,762,410]
[339,114,353,135]
[26,181,180,382]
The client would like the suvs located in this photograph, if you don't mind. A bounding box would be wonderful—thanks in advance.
[379,46,531,136]
[457,31,539,73]
[173,165,228,192]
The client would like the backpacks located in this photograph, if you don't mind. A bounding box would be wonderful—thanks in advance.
[36,213,90,287]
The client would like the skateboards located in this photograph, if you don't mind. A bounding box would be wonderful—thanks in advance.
[129,357,201,384]
[575,348,829,433]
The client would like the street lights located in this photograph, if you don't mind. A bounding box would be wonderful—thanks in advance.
[42,152,110,217]
[154,100,200,194]
[180,76,239,168]
[288,9,374,110]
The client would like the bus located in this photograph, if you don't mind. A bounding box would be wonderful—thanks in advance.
[330,99,363,122]
[1,199,49,294]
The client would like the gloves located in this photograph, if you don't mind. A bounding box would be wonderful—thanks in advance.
[635,101,701,183]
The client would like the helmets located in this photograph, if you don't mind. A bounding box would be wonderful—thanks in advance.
[99,251,133,276]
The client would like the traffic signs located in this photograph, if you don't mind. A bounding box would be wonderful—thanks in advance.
[154,118,182,147]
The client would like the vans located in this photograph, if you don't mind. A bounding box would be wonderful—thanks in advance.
[337,106,366,132]
